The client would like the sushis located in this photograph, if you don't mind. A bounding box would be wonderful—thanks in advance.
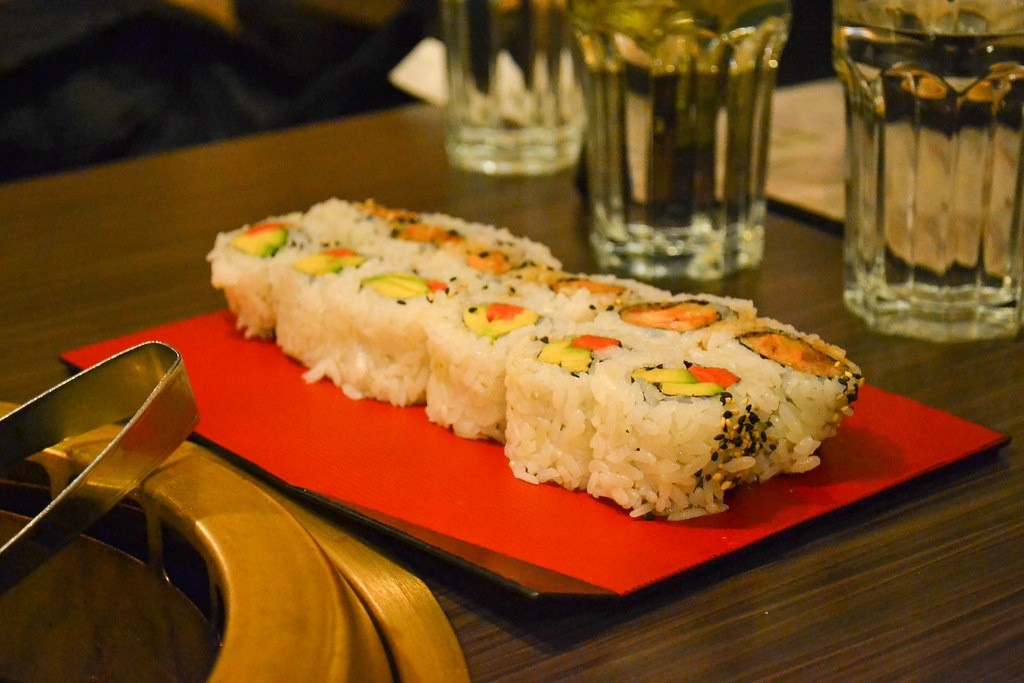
[205,198,866,521]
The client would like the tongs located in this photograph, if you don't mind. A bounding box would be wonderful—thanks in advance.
[0,339,198,596]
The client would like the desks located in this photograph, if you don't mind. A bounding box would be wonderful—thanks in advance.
[0,101,1024,683]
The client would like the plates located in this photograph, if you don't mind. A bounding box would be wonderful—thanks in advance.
[59,285,1012,629]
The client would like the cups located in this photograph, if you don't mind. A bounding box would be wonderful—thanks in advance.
[438,0,589,178]
[833,0,1024,344]
[569,0,792,287]
